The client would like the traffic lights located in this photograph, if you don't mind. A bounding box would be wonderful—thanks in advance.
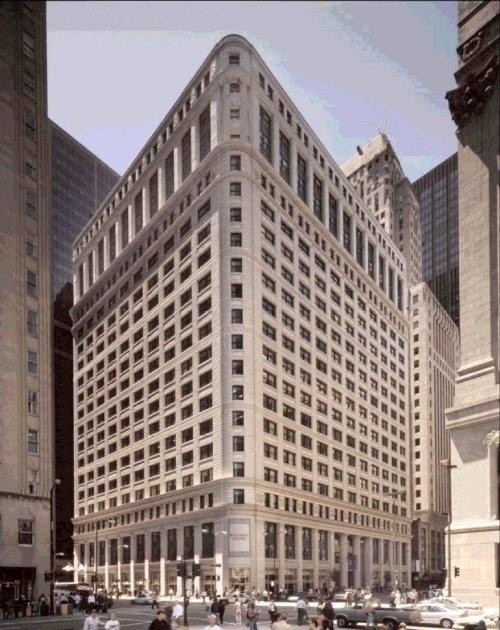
[176,562,184,577]
[191,563,201,577]
[441,568,447,578]
[454,566,459,577]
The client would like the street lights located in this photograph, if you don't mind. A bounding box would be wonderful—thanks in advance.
[52,553,66,615]
[93,519,115,591]
[115,543,129,596]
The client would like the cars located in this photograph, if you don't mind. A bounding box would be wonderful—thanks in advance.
[216,593,255,605]
[131,593,157,605]
[329,591,485,629]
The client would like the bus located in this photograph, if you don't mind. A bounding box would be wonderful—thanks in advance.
[51,580,90,600]
